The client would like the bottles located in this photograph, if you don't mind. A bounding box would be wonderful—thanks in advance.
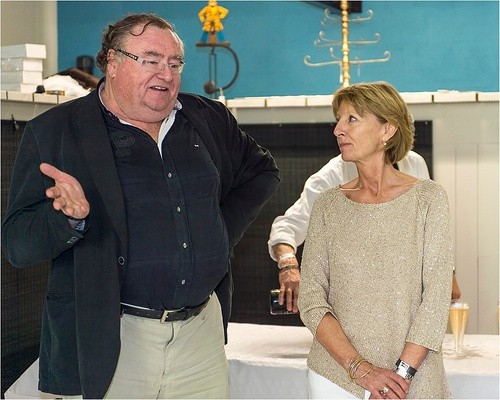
[270,289,299,315]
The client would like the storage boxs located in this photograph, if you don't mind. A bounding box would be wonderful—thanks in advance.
[0,44,47,93]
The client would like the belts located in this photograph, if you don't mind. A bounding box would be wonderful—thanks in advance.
[119,291,214,324]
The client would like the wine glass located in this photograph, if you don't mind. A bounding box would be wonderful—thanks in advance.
[450,298,470,357]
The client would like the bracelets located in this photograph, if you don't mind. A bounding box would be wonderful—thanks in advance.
[278,264,300,275]
[394,359,416,380]
[347,354,373,381]
[278,253,296,260]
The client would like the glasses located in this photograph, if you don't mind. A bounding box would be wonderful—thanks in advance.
[114,48,185,74]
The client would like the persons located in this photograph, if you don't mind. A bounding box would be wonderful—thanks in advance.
[269,147,460,314]
[296,81,453,400]
[0,13,278,400]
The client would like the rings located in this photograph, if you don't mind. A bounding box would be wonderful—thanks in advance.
[381,386,389,395]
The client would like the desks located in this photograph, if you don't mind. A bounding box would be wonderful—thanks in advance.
[4,322,499,398]
